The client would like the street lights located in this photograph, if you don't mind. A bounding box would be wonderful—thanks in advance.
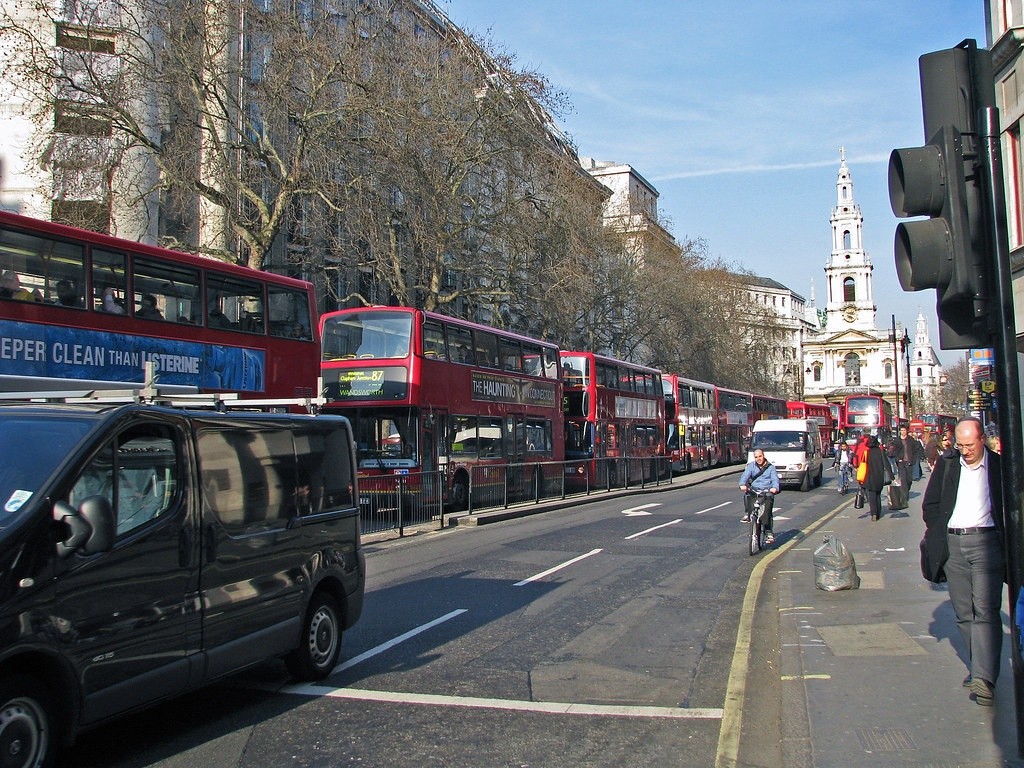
[901,326,914,410]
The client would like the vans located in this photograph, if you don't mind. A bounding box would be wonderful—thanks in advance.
[0,393,367,767]
[743,419,824,492]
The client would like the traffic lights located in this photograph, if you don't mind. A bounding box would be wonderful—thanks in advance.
[887,123,973,311]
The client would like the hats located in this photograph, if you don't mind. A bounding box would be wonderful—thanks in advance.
[866,436,880,447]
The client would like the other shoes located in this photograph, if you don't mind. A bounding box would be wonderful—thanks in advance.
[963,674,971,686]
[740,517,750,522]
[970,677,994,706]
[872,515,879,521]
[764,532,774,542]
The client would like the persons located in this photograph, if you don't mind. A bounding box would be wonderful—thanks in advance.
[852,427,872,509]
[456,345,474,364]
[863,436,896,521]
[501,352,509,369]
[788,435,803,447]
[136,295,164,320]
[97,287,125,313]
[291,322,302,338]
[739,448,779,541]
[834,442,853,491]
[210,309,231,328]
[922,413,1005,706]
[892,426,955,501]
[54,280,85,308]
[238,311,261,333]
[2,271,33,301]
[988,435,1000,455]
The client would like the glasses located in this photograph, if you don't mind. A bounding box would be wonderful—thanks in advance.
[942,438,948,441]
[954,438,980,449]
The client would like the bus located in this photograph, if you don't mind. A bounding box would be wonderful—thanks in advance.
[891,416,909,439]
[844,394,892,453]
[751,394,787,432]
[310,306,566,512]
[618,374,720,473]
[916,413,958,439]
[1,209,325,415]
[785,401,832,458]
[517,350,667,491]
[715,387,750,465]
[826,403,846,458]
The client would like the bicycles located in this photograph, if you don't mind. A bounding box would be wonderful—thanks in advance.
[741,486,775,556]
[833,463,857,496]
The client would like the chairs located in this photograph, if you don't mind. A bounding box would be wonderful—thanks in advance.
[425,350,438,359]
[343,353,356,359]
[480,360,490,366]
[465,354,474,364]
[99,464,162,533]
[359,353,374,358]
[492,362,499,367]
[440,353,452,360]
[504,364,513,369]
[453,355,466,363]
[183,319,198,325]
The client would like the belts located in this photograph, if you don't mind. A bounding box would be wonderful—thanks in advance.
[948,527,995,535]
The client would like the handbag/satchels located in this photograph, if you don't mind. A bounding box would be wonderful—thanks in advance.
[746,478,753,488]
[856,451,866,481]
[920,541,932,580]
[883,468,891,485]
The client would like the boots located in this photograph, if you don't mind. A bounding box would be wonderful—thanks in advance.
[855,494,864,508]
